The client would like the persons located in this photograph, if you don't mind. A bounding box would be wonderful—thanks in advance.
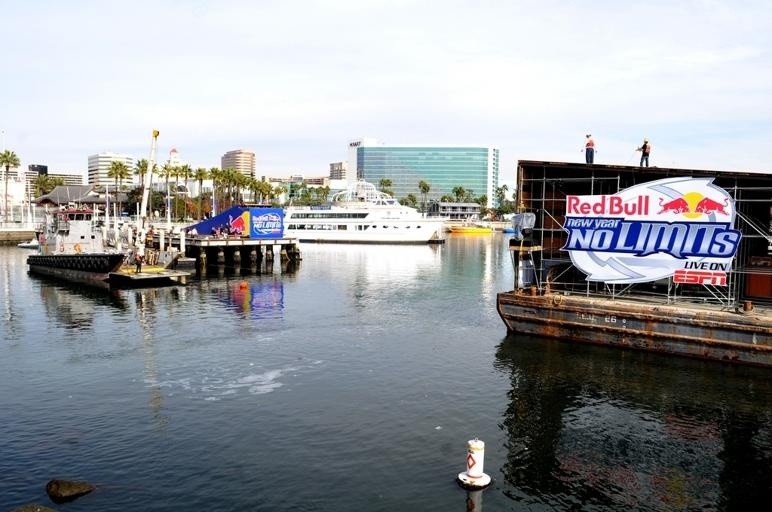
[580,132,600,166]
[633,136,653,169]
[133,252,145,273]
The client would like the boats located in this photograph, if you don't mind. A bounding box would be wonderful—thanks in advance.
[503,228,515,233]
[448,225,492,233]
[26,186,190,292]
[283,171,446,244]
[17,238,39,248]
